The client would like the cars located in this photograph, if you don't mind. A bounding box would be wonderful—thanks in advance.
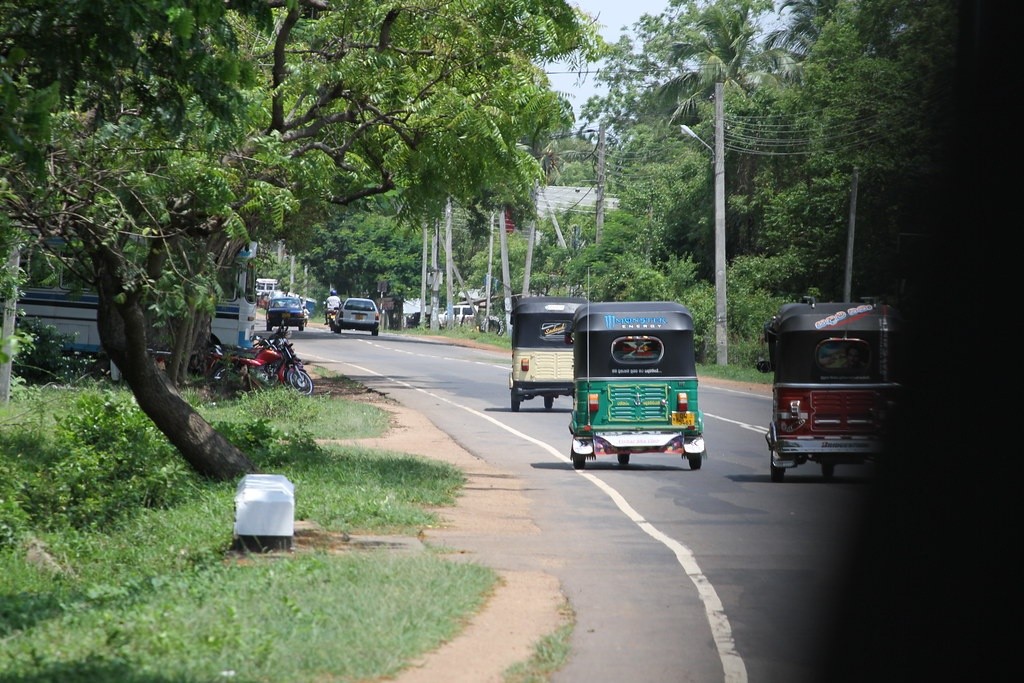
[334,297,382,336]
[264,297,306,332]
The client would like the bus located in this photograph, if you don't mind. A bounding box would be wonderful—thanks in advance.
[1,230,257,378]
[256,278,282,308]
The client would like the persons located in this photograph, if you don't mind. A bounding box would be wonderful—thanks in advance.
[303,306,310,327]
[324,290,341,325]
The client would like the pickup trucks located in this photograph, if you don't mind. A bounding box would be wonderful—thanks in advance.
[438,303,486,330]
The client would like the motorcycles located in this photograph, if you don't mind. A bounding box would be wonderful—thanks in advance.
[202,320,313,400]
[756,296,902,483]
[508,298,591,412]
[564,300,707,470]
[323,300,337,331]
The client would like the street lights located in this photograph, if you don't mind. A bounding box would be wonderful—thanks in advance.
[680,124,728,366]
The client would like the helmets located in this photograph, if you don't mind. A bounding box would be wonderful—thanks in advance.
[331,290,336,295]
